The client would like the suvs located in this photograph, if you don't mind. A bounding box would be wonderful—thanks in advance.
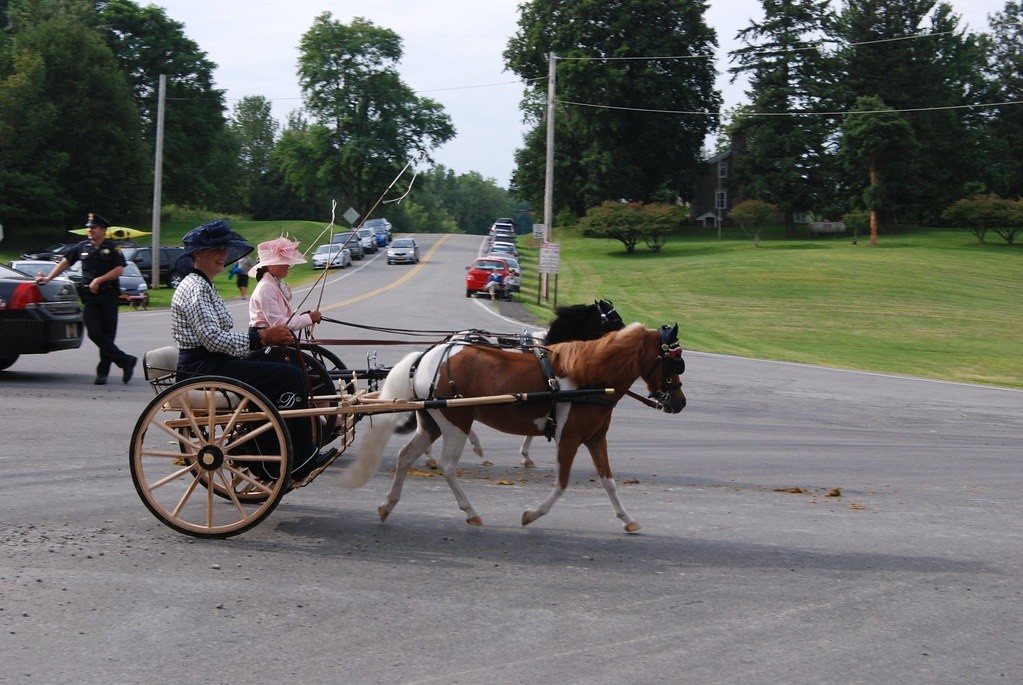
[119,247,186,289]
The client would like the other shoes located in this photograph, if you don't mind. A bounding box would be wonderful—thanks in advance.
[95,376,107,385]
[123,355,137,384]
[334,414,344,428]
[242,297,245,300]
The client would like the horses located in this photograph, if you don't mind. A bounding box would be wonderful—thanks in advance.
[340,297,687,533]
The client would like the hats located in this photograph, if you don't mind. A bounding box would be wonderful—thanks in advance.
[247,237,307,278]
[174,219,255,276]
[86,213,111,227]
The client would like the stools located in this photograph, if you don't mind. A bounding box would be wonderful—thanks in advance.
[128,295,148,310]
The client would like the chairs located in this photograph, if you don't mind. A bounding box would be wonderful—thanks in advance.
[142,346,246,409]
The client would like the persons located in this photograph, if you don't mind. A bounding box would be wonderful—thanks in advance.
[171,222,349,481]
[235,256,255,301]
[484,269,520,302]
[34,213,137,385]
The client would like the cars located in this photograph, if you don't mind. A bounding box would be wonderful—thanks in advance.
[311,243,353,270]
[6,260,74,283]
[483,256,522,293]
[327,231,364,261]
[385,238,420,266]
[464,257,515,299]
[364,219,392,246]
[0,263,84,369]
[18,242,78,260]
[486,217,520,262]
[352,227,378,255]
[117,260,150,305]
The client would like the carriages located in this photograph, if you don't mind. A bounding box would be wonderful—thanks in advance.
[127,300,688,541]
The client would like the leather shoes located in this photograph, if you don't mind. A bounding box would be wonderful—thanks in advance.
[294,447,338,482]
